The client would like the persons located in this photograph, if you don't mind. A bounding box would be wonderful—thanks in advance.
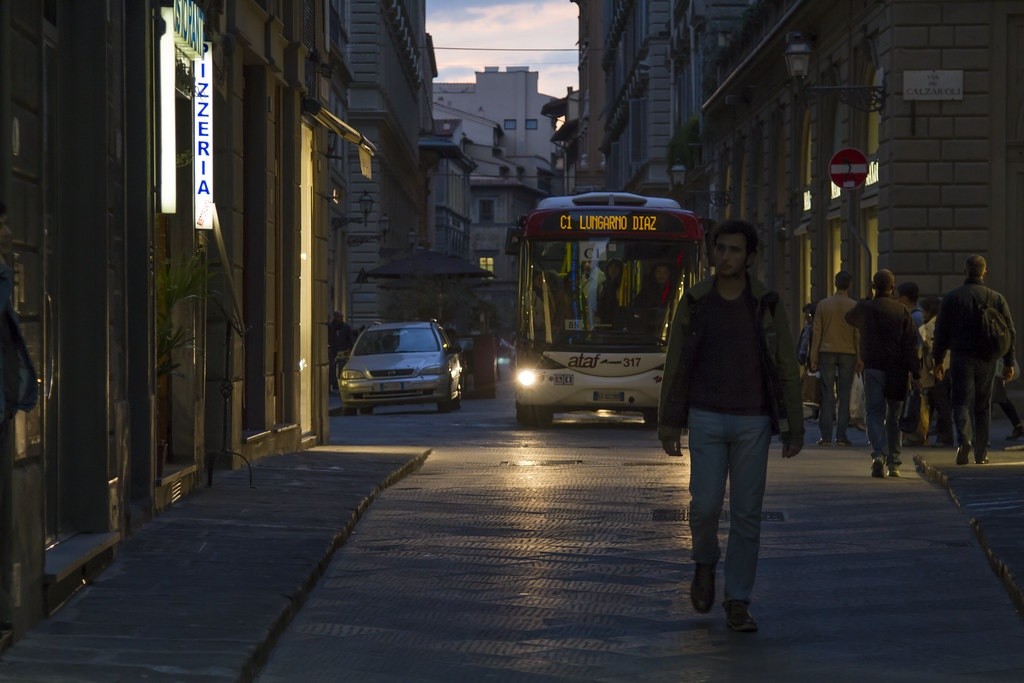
[795,254,1024,477]
[658,221,805,632]
[328,305,517,396]
[532,260,675,332]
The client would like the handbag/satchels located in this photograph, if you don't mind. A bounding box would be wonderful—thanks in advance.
[898,388,921,433]
[801,368,822,408]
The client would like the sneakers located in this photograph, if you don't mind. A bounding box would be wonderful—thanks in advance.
[690,550,722,614]
[722,597,758,631]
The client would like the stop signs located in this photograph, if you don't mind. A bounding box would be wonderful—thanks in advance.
[828,147,868,190]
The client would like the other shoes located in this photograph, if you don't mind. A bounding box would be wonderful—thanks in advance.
[870,455,885,479]
[1005,424,1024,441]
[931,441,953,449]
[821,435,832,446]
[834,437,852,446]
[956,442,971,464]
[888,470,900,477]
[975,456,989,464]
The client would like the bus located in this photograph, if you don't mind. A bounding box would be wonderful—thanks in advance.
[504,190,717,422]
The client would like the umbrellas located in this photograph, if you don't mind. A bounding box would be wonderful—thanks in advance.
[367,252,497,321]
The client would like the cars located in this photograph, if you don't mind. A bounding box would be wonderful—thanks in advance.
[338,319,463,416]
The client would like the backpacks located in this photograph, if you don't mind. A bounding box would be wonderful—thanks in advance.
[963,284,1011,363]
